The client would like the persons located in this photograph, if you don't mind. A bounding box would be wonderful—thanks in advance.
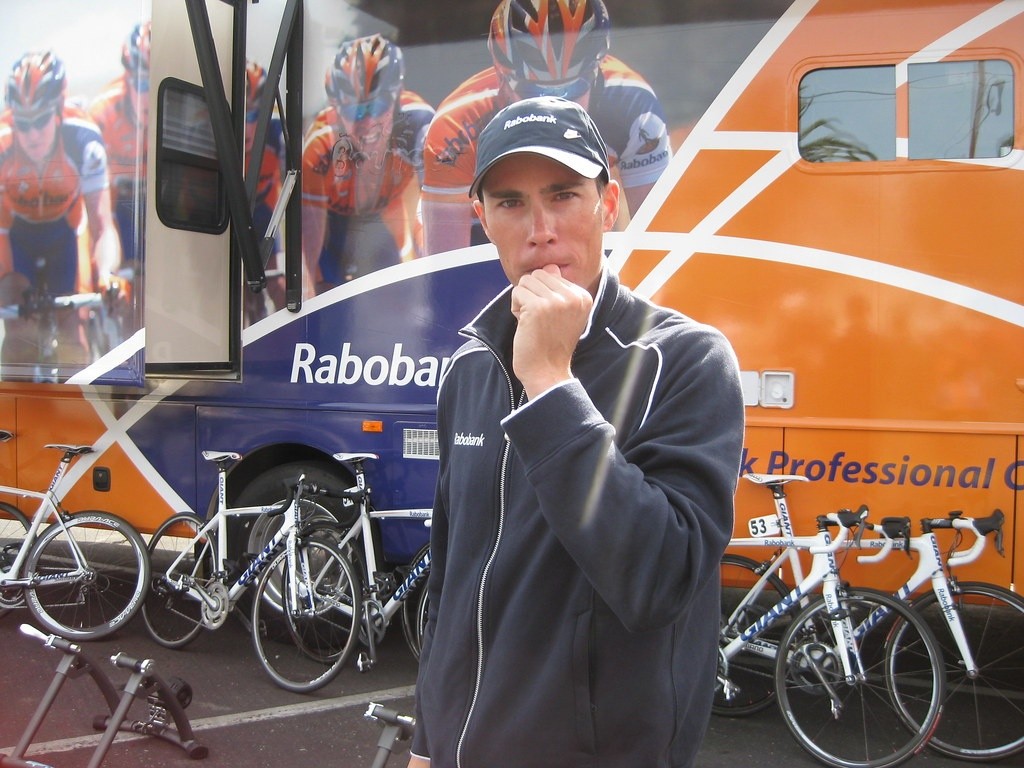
[409,95,745,768]
[0,0,674,382]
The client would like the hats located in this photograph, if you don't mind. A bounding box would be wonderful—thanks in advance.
[469,96,610,198]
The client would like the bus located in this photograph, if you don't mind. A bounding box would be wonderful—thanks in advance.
[0,0,1024,653]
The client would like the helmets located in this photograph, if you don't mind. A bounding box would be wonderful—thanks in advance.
[128,76,149,92]
[486,0,611,89]
[245,58,268,108]
[4,52,67,117]
[121,19,151,73]
[325,34,405,105]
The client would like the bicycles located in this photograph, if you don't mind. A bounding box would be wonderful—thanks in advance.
[282,452,432,667]
[720,474,1024,768]
[140,450,372,694]
[0,432,150,640]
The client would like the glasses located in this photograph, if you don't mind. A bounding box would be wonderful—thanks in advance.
[500,65,600,106]
[13,105,57,131]
[244,108,263,124]
[335,86,398,121]
